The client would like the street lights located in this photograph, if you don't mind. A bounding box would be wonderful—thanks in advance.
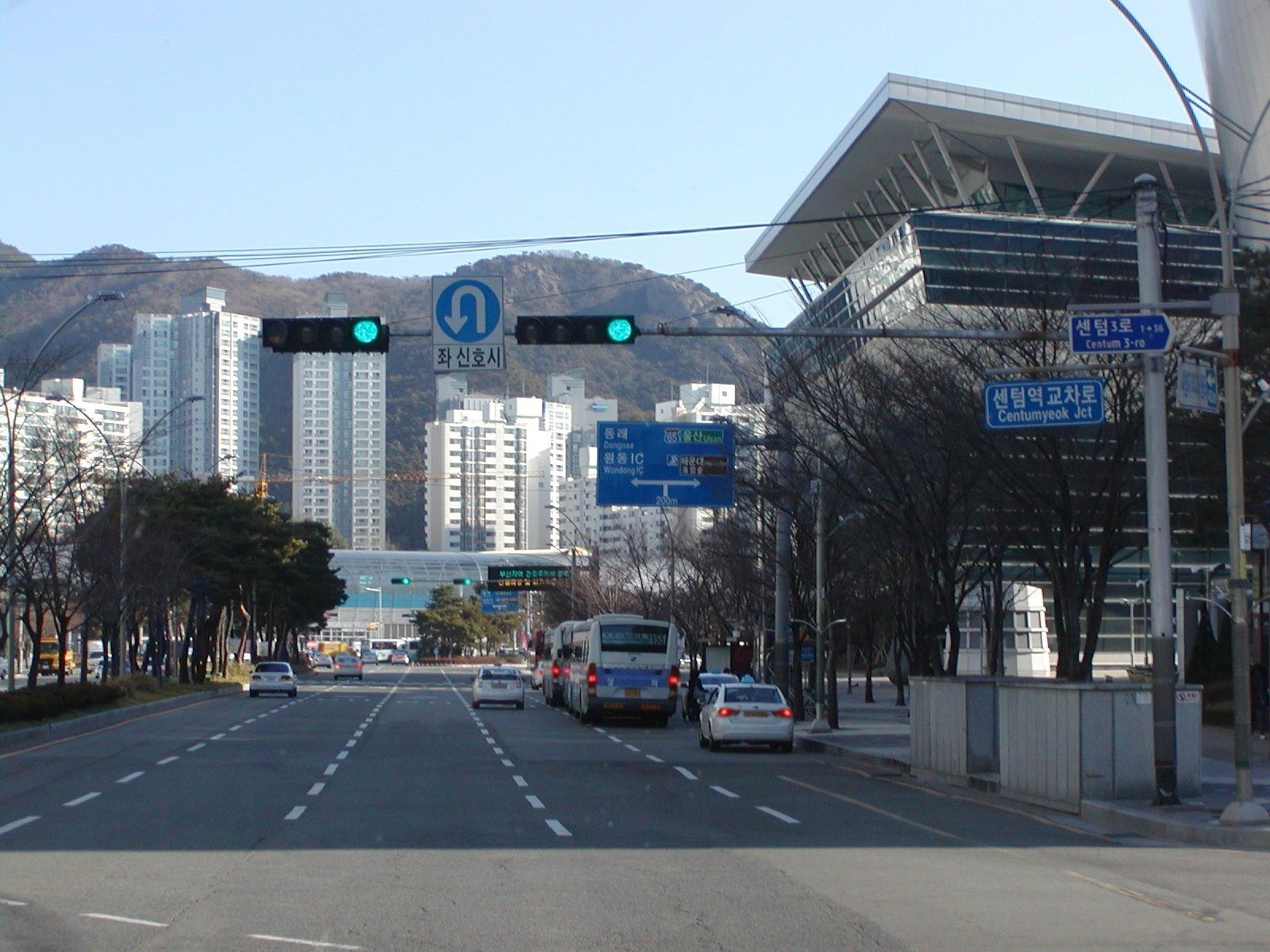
[707,303,830,732]
[42,395,208,680]
[1135,579,1150,669]
[0,289,127,693]
[1120,597,1145,670]
[365,586,383,639]
[543,503,600,622]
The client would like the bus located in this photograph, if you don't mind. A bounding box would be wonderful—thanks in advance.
[557,614,680,725]
[526,629,548,670]
[360,636,422,665]
[538,619,587,707]
[38,636,77,676]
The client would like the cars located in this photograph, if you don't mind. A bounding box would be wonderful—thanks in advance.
[391,651,409,665]
[529,662,546,689]
[333,653,364,680]
[248,661,298,698]
[297,640,362,669]
[0,655,9,681]
[681,672,796,753]
[87,637,251,680]
[469,664,525,709]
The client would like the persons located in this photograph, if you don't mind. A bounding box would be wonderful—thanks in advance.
[723,661,757,684]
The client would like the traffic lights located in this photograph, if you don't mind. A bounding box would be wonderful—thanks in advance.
[390,578,411,586]
[452,576,473,586]
[515,314,638,345]
[259,315,385,353]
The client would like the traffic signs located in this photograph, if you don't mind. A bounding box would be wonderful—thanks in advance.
[592,420,739,510]
